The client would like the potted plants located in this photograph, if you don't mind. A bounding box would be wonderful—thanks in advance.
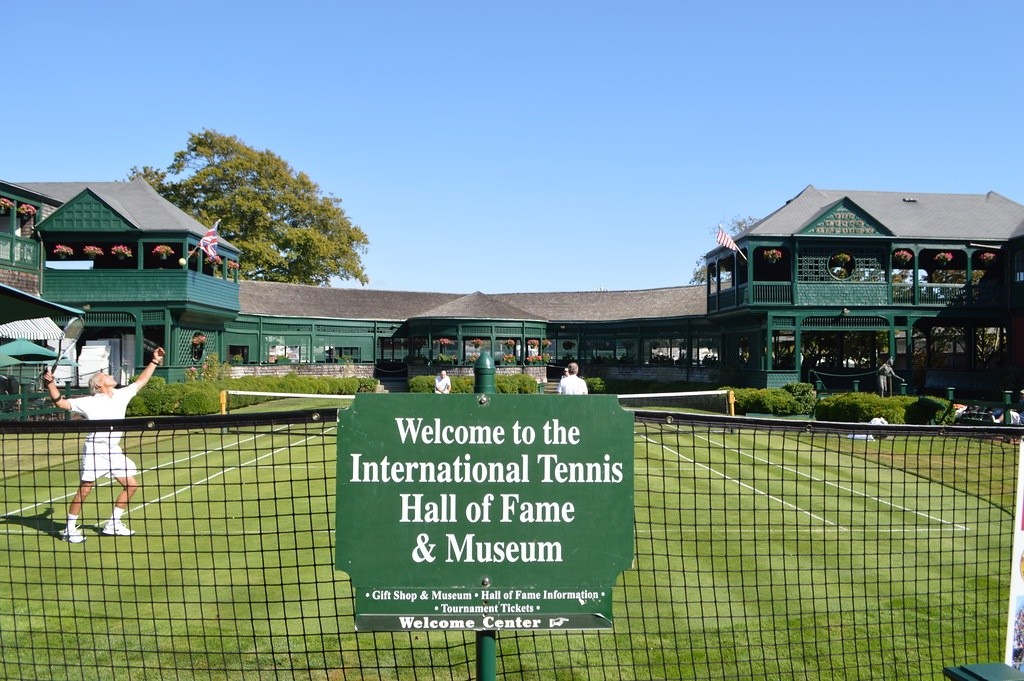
[834,253,850,267]
[233,355,243,364]
[276,355,291,364]
[343,355,352,364]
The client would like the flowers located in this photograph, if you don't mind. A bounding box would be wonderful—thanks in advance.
[83,246,104,255]
[53,244,73,255]
[152,244,174,254]
[763,249,781,260]
[979,252,995,261]
[934,252,953,262]
[110,245,133,257]
[204,255,222,265]
[403,339,552,366]
[228,261,240,270]
[192,335,206,344]
[17,203,37,214]
[0,198,13,209]
[894,250,912,261]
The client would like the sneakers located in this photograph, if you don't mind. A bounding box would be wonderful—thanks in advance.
[102,520,135,536]
[62,525,87,543]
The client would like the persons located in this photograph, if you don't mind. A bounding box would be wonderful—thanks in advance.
[434,370,451,394]
[44,347,165,543]
[561,368,569,379]
[988,389,1024,426]
[556,363,588,395]
[878,358,905,398]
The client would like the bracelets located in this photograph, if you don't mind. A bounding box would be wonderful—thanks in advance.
[151,360,159,366]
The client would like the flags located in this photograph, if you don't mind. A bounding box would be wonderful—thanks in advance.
[197,221,218,262]
[716,229,737,252]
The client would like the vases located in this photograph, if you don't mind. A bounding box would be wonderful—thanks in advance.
[0,208,6,215]
[939,260,946,266]
[768,258,776,264]
[22,213,30,220]
[58,253,167,260]
[196,343,200,349]
[899,259,906,265]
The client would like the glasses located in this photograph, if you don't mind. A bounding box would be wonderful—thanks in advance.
[97,373,108,385]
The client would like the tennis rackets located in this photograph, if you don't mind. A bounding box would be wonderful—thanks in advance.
[44,317,84,387]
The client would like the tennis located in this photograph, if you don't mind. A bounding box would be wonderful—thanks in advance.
[179,258,186,266]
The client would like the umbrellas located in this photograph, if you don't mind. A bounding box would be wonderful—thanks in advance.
[0,340,82,383]
